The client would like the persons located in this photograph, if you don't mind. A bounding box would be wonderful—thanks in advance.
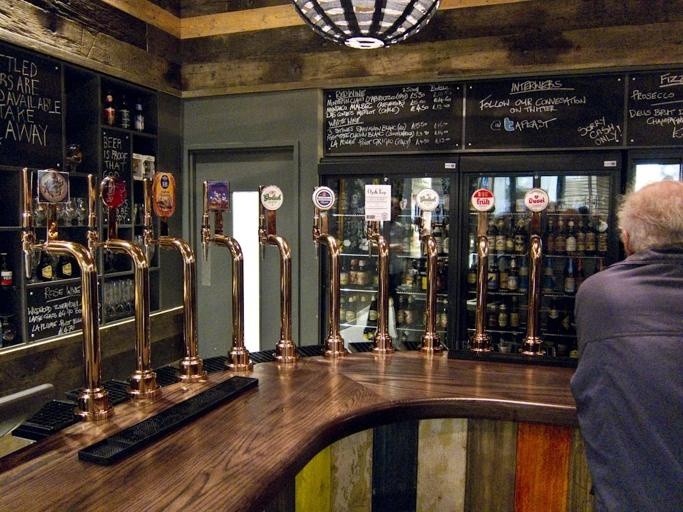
[571,177,683,511]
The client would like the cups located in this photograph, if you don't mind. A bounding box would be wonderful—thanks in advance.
[133,234,144,246]
[32,196,88,227]
[103,278,135,317]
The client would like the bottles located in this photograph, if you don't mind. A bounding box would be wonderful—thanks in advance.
[58,252,73,279]
[0,251,14,288]
[38,240,52,281]
[331,178,610,359]
[104,88,146,133]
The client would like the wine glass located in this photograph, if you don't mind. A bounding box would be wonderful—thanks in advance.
[138,203,145,225]
[65,143,83,173]
[1,313,17,343]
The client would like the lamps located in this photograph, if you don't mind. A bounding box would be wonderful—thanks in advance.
[291,0,441,50]
